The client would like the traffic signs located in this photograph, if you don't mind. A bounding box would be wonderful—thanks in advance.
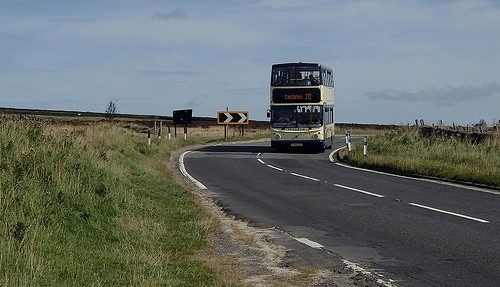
[217,110,249,125]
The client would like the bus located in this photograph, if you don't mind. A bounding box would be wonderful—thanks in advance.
[267,62,334,153]
